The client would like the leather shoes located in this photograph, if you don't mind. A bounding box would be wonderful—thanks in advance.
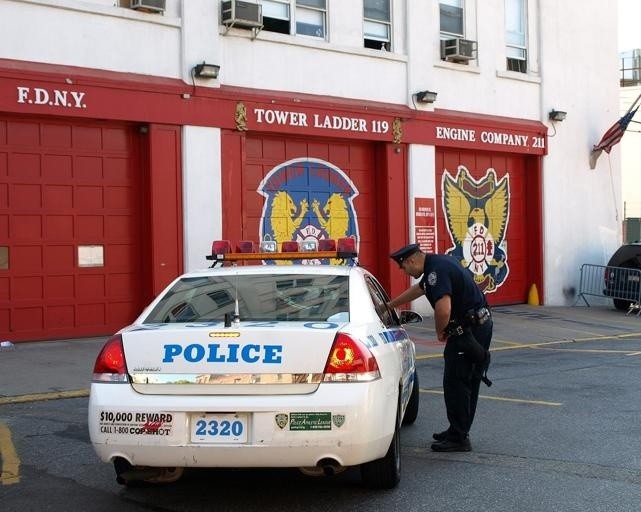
[431,424,472,452]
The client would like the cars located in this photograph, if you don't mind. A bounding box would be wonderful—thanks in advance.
[85,233,425,488]
[601,240,641,317]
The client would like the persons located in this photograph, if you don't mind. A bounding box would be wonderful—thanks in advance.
[386,244,493,452]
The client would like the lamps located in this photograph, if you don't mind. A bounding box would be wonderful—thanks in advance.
[195,63,220,78]
[417,91,437,103]
[550,111,567,121]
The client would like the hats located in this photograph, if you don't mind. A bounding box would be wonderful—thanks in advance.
[389,244,418,268]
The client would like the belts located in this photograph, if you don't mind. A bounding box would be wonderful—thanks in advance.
[472,308,490,324]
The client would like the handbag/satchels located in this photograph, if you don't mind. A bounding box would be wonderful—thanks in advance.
[444,321,489,362]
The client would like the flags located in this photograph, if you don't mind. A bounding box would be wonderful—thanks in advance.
[593,109,637,153]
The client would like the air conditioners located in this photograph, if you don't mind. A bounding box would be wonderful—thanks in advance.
[222,0,260,25]
[441,39,473,60]
[130,0,166,13]
